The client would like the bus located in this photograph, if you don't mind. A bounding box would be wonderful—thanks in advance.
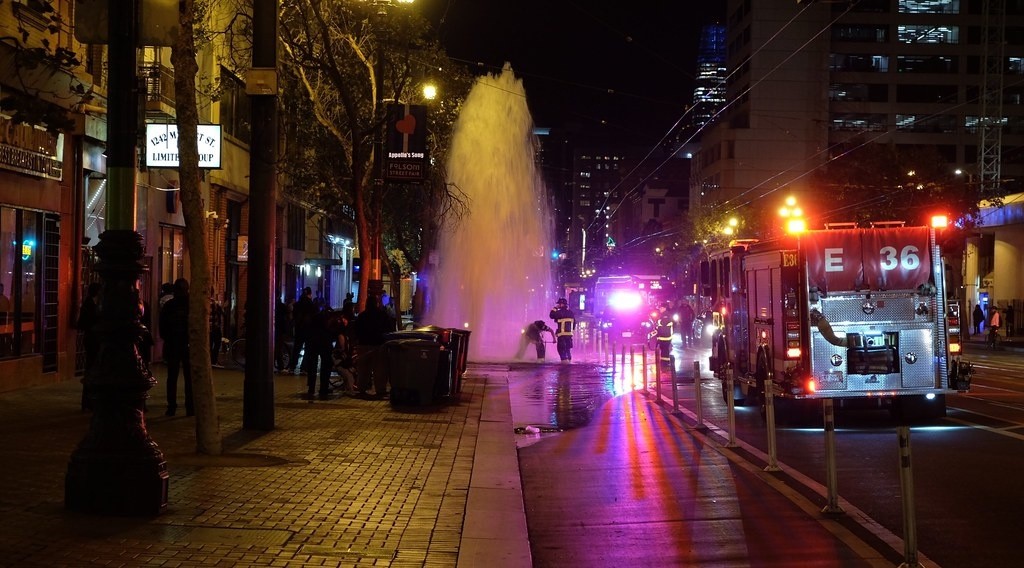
[595,275,677,318]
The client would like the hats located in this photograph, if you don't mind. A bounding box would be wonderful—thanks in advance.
[536,321,546,330]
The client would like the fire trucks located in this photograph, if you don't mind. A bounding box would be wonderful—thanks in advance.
[698,221,976,420]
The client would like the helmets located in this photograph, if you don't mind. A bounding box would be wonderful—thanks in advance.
[556,298,567,304]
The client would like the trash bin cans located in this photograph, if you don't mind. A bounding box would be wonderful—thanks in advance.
[384,326,472,411]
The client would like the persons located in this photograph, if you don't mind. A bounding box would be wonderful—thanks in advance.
[1005,305,1015,337]
[972,304,983,335]
[656,303,673,367]
[78,282,101,412]
[516,320,556,359]
[677,298,695,347]
[550,299,576,361]
[211,286,224,368]
[275,287,396,400]
[158,278,194,417]
[988,306,1003,347]
[665,301,676,314]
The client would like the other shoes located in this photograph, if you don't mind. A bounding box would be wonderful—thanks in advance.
[537,358,545,363]
[165,404,177,416]
[186,407,196,417]
[562,359,570,364]
[280,368,294,375]
[369,392,384,400]
[212,364,225,368]
[300,366,307,375]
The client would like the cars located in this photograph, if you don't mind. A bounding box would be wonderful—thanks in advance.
[595,300,658,348]
[691,307,711,348]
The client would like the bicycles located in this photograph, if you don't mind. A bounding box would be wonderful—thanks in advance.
[983,325,1003,351]
